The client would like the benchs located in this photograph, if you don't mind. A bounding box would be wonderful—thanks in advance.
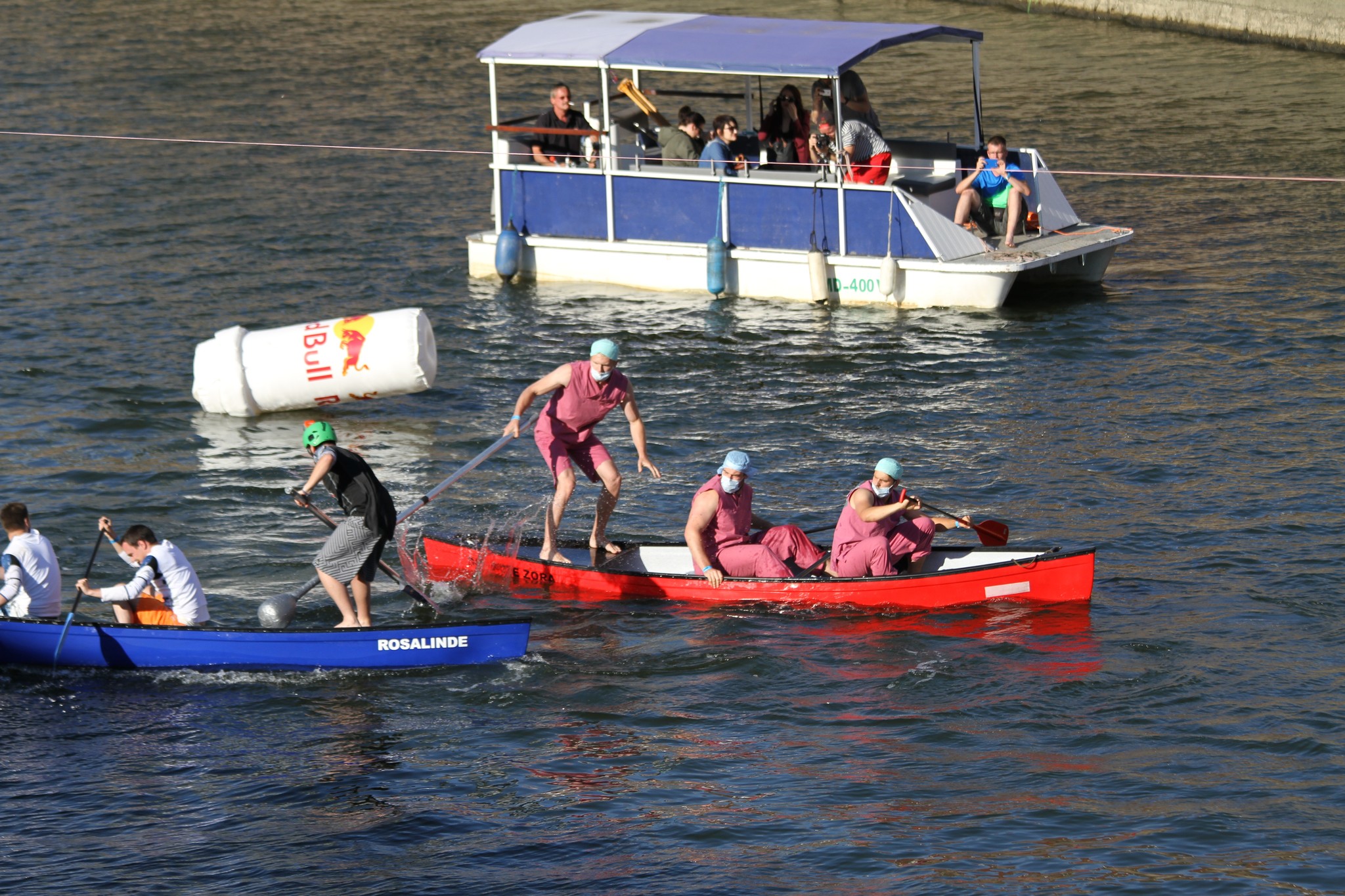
[884,138,962,222]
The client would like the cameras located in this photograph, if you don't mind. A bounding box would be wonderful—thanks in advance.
[819,89,831,96]
[814,133,830,148]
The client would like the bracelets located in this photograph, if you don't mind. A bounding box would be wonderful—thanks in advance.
[301,488,311,496]
[702,566,714,573]
[956,519,961,529]
[108,535,120,544]
[510,415,521,421]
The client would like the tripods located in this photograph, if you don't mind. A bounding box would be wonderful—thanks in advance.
[814,154,831,173]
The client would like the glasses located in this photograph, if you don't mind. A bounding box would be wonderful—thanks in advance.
[723,125,738,132]
[781,95,794,103]
[696,127,704,135]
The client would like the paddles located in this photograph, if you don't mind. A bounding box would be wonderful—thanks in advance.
[899,488,1010,547]
[54,517,109,660]
[617,77,673,128]
[804,522,838,535]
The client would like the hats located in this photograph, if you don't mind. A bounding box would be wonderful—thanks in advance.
[875,458,903,480]
[716,451,758,479]
[817,110,836,128]
[590,339,620,361]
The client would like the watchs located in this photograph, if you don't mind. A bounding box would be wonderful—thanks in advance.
[826,148,834,157]
[842,96,849,106]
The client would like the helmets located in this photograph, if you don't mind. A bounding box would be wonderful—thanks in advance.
[301,422,338,447]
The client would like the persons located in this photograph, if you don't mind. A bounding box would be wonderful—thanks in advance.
[294,422,397,629]
[0,502,62,620]
[532,82,599,168]
[809,111,892,185]
[661,105,752,177]
[75,515,211,628]
[826,457,974,576]
[954,135,1031,248]
[502,338,662,564]
[758,83,811,171]
[811,69,883,140]
[682,450,839,590]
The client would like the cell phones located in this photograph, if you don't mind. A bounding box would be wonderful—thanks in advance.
[983,159,998,168]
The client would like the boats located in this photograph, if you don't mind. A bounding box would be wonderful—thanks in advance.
[0,602,536,670]
[422,523,1099,608]
[462,8,1135,310]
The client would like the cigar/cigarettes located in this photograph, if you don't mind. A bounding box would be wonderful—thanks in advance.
[568,101,575,106]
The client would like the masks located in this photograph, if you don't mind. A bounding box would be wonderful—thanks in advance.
[590,367,611,382]
[871,482,894,498]
[721,471,744,494]
